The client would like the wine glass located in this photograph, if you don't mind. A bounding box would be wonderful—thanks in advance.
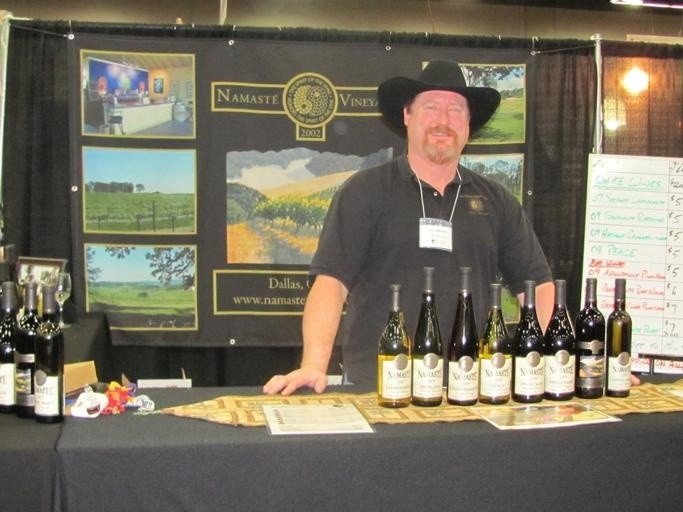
[55,274,72,329]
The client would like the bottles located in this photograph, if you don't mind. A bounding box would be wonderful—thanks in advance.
[377,264,633,409]
[0,279,64,424]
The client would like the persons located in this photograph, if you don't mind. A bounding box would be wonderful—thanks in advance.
[261,60,556,395]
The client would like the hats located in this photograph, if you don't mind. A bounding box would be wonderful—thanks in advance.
[377,60,500,137]
